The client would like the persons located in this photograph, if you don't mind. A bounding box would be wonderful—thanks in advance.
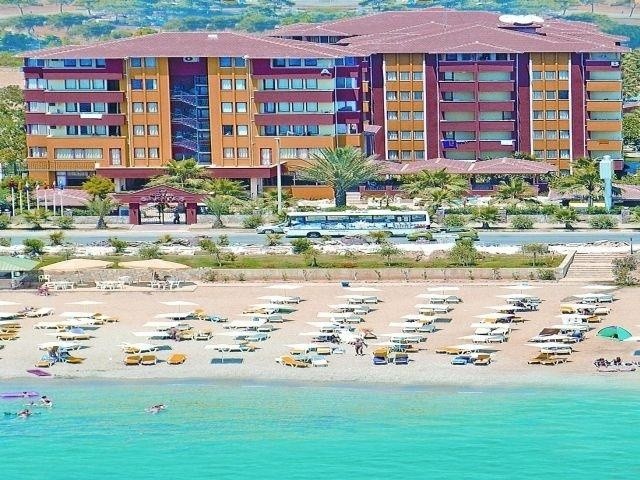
[15,390,53,427]
[594,356,624,367]
[171,209,182,225]
[146,401,168,413]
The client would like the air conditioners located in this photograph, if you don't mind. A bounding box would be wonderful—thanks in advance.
[183,57,199,63]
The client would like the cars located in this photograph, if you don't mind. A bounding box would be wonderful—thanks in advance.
[255,222,283,235]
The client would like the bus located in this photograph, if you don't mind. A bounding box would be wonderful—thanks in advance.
[282,210,431,239]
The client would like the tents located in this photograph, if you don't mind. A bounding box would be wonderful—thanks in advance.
[596,325,632,342]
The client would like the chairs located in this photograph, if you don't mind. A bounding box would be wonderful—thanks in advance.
[367,177,398,190]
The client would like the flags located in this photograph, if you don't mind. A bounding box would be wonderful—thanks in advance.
[9,178,66,196]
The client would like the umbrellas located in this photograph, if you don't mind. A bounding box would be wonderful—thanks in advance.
[117,257,190,284]
[38,258,115,287]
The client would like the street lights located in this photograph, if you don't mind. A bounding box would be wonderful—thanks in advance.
[273,137,282,219]
